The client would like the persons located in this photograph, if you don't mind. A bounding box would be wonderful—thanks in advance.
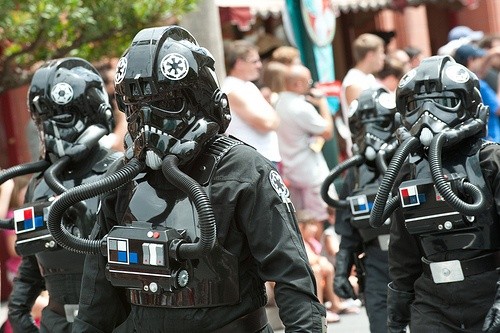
[0,25,500,333]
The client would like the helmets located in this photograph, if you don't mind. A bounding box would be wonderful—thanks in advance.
[115,26,231,162]
[27,57,113,161]
[394,55,489,146]
[347,87,398,161]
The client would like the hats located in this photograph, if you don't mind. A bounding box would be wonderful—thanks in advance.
[454,45,485,62]
[447,25,484,41]
[437,37,471,56]
